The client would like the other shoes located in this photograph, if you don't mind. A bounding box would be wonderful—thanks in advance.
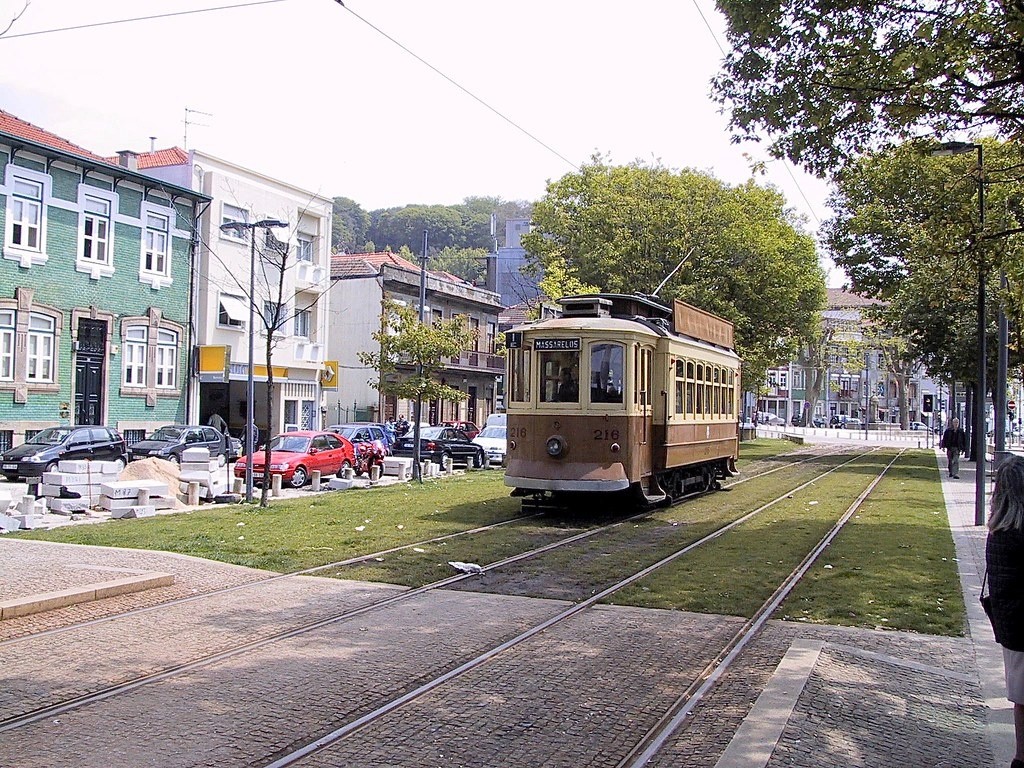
[1010,758,1024,768]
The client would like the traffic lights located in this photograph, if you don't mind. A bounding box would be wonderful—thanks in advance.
[924,395,932,411]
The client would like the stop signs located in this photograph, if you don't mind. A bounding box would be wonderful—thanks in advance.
[1007,400,1016,410]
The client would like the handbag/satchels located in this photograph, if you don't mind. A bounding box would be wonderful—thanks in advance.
[980,566,1001,645]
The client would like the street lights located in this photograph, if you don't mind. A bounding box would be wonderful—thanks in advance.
[925,143,988,527]
[221,219,288,505]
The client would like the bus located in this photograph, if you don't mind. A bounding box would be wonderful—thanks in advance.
[504,288,740,518]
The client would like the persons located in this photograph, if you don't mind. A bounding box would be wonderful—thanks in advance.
[386,415,397,444]
[406,415,415,432]
[239,418,260,454]
[941,417,967,479]
[983,454,1024,768]
[394,414,410,437]
[738,406,840,428]
[553,367,576,402]
[207,409,228,431]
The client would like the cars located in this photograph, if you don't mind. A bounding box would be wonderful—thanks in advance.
[0,425,129,481]
[392,426,485,470]
[318,420,396,458]
[738,411,1023,437]
[232,432,359,489]
[131,425,243,469]
[435,412,509,466]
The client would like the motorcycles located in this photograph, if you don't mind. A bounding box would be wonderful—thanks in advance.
[355,434,386,480]
